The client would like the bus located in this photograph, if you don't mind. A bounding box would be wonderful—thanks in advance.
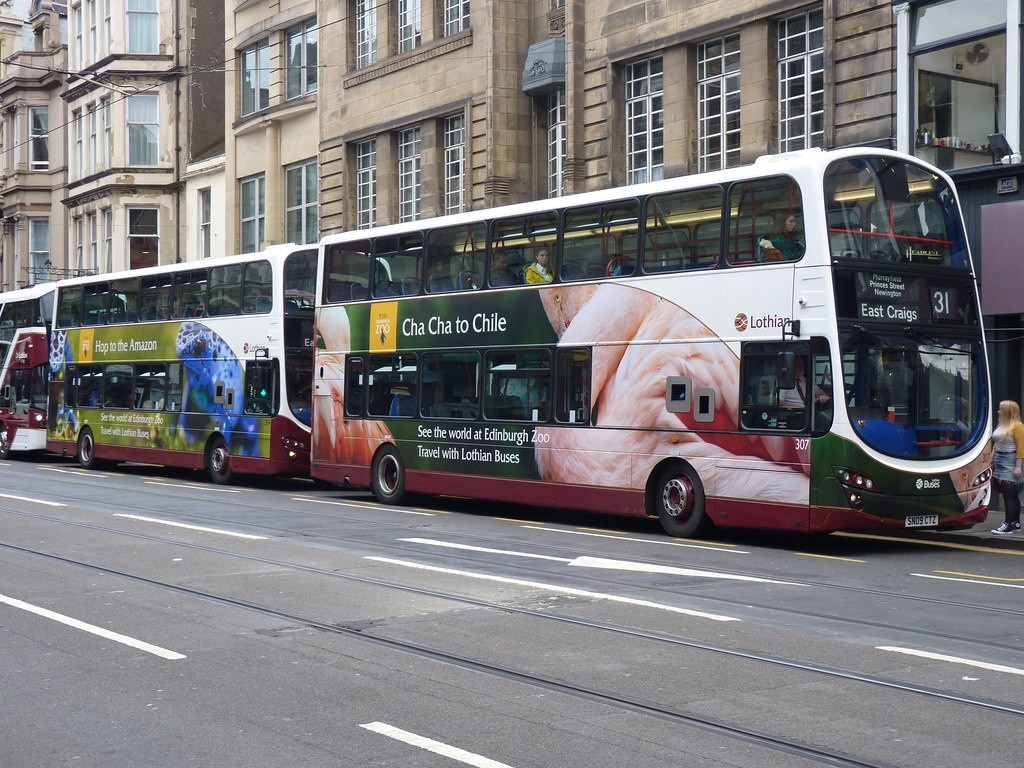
[0,280,129,461]
[309,144,994,538]
[45,242,394,485]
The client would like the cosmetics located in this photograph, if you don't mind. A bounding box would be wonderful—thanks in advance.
[922,128,986,152]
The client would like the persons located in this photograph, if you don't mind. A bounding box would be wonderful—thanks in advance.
[771,208,804,259]
[142,398,171,410]
[490,251,519,282]
[524,246,555,284]
[990,400,1024,536]
[767,355,829,409]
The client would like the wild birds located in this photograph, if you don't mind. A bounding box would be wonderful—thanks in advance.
[534,283,810,504]
[315,307,397,466]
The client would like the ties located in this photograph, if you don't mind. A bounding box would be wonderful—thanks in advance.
[796,377,805,404]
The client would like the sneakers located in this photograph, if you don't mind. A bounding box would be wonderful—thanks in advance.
[991,522,1014,535]
[1009,522,1022,532]
